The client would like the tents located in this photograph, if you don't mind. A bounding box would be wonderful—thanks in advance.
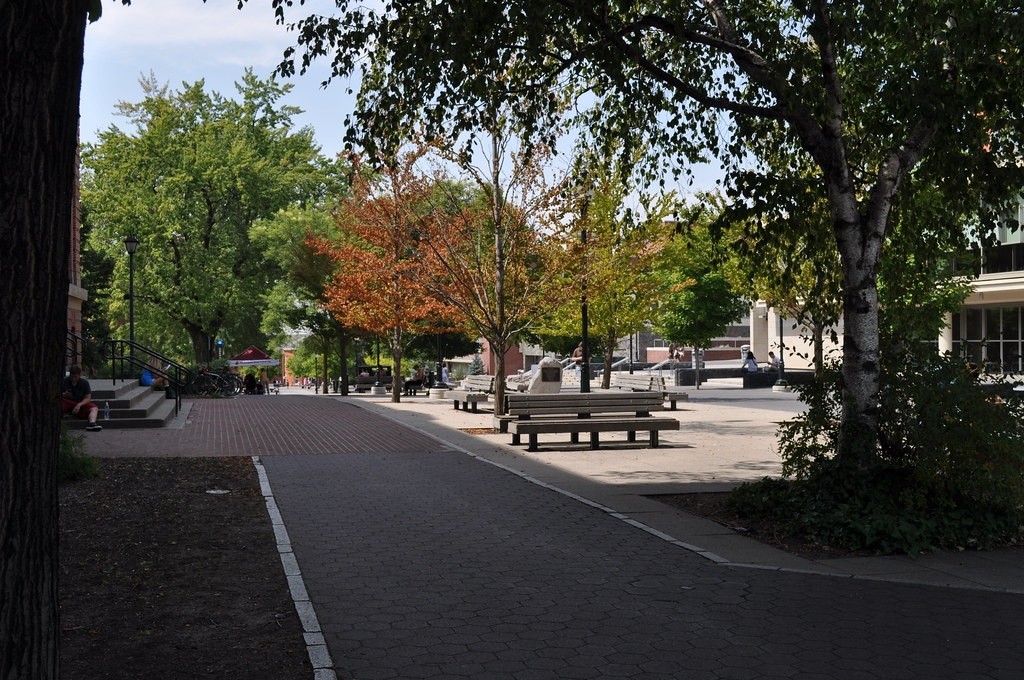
[226,344,281,396]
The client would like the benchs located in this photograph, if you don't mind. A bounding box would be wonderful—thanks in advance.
[444,375,493,413]
[354,376,404,393]
[493,394,652,433]
[742,372,816,388]
[508,392,680,452]
[675,368,762,386]
[615,373,688,411]
[506,382,529,392]
[402,371,434,396]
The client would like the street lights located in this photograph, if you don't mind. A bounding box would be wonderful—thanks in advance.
[123,232,141,379]
[353,334,362,391]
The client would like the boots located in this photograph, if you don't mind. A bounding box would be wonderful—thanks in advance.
[164,386,176,399]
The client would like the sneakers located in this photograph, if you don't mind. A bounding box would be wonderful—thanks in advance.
[85,422,103,432]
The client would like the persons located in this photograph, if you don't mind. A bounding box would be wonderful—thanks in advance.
[58,364,102,432]
[741,351,758,378]
[765,351,781,373]
[286,359,451,396]
[140,349,182,400]
[242,366,270,396]
[572,341,592,377]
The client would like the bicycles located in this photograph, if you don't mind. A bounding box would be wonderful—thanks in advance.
[190,363,244,396]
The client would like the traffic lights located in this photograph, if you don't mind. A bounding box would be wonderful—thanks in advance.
[218,340,222,349]
